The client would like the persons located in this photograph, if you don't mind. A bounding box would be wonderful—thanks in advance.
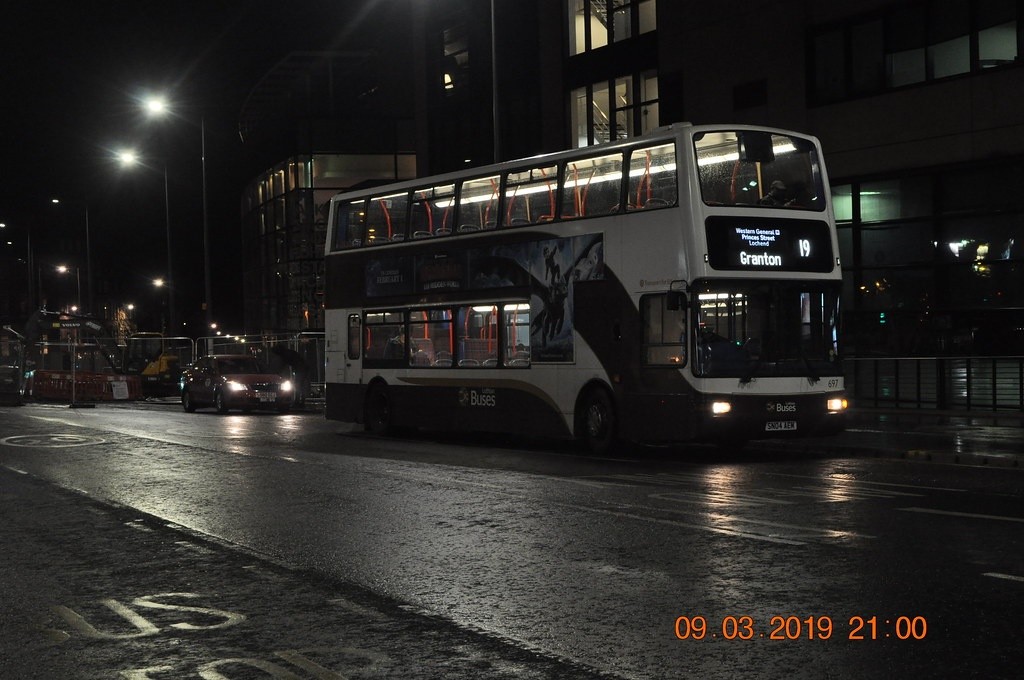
[381,324,419,360]
[270,344,309,407]
[760,181,793,206]
[541,242,561,286]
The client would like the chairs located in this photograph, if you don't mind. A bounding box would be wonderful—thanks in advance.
[352,198,670,249]
[433,350,531,367]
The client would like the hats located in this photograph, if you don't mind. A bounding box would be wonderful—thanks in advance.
[771,181,786,190]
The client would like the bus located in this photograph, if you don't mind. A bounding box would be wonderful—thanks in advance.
[324,120,852,458]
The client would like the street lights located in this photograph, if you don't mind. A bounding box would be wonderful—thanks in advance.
[111,141,175,337]
[138,92,215,338]
[50,198,92,312]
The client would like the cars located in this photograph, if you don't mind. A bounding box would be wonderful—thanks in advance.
[180,354,291,415]
